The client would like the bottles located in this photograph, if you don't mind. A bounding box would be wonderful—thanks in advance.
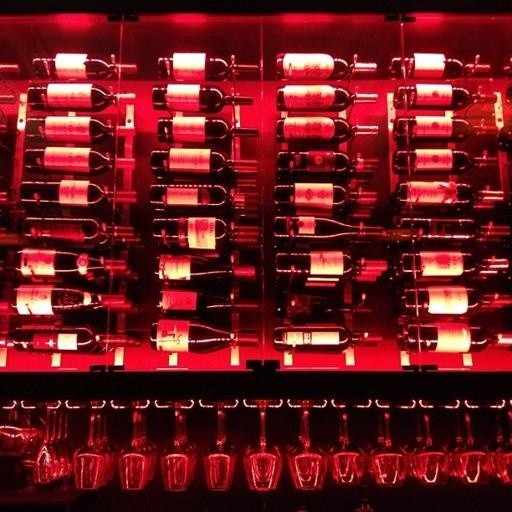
[0,52,512,356]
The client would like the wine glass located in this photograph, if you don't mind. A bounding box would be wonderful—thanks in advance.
[0,401,512,492]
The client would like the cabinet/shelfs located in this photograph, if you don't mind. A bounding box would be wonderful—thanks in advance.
[0,1,512,511]
